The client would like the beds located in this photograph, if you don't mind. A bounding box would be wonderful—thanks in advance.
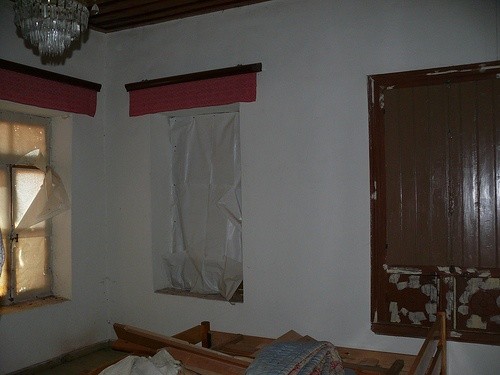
[87,311,450,374]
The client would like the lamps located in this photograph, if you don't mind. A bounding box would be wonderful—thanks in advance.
[12,0,100,60]
